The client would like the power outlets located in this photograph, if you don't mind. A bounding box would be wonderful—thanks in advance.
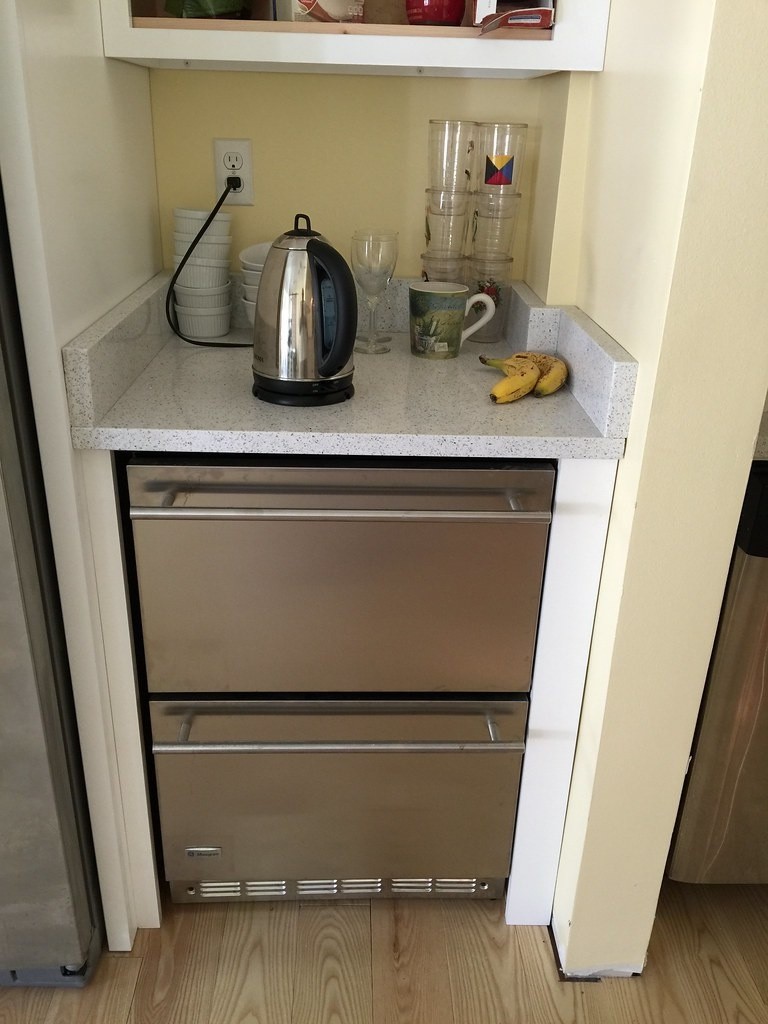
[213,138,255,207]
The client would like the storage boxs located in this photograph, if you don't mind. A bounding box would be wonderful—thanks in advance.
[473,0,554,36]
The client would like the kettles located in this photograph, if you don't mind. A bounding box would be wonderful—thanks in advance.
[251,213,359,408]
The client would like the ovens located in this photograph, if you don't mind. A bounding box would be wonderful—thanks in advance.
[123,460,557,906]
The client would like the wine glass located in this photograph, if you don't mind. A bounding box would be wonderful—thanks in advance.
[350,229,399,355]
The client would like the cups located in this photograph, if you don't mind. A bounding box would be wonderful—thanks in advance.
[408,281,495,361]
[419,121,529,342]
[406,0,466,27]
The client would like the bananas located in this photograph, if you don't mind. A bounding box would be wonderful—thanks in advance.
[479,351,568,404]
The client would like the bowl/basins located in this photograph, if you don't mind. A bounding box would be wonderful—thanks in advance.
[169,206,274,340]
